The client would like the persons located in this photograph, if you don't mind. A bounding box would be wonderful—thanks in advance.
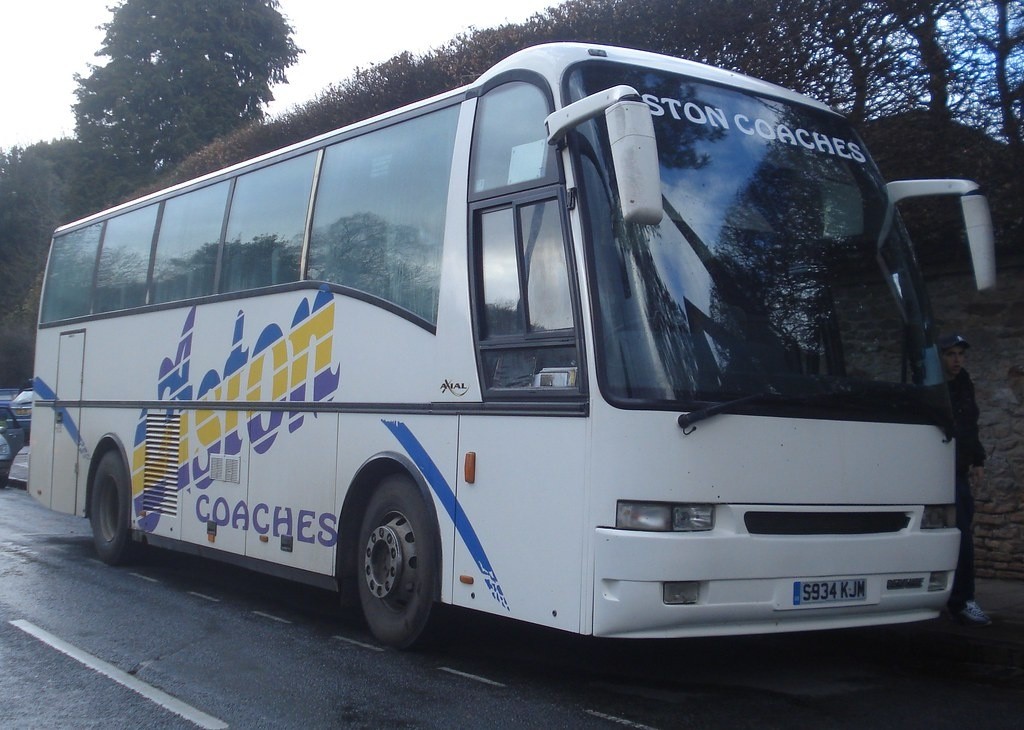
[942,326,991,624]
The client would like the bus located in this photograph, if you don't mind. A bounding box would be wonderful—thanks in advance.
[25,41,997,652]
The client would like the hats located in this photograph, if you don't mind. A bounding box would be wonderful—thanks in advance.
[934,333,971,356]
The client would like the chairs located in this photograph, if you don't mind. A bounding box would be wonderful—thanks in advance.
[120,239,290,307]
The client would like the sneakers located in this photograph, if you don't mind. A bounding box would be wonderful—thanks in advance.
[948,595,992,628]
